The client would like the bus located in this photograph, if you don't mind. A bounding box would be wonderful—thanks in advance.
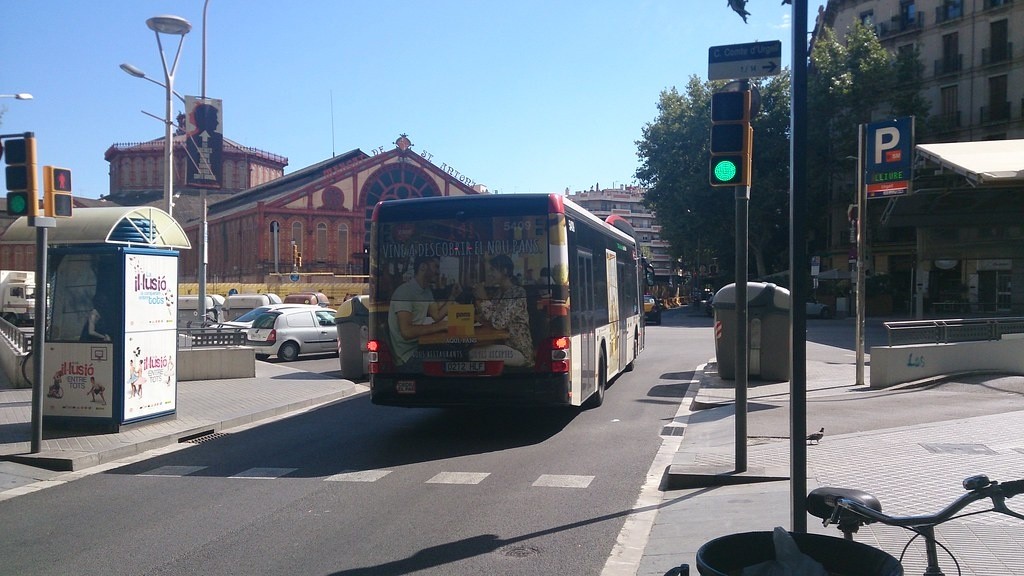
[366,193,655,412]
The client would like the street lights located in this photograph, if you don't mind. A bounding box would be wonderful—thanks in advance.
[120,62,209,332]
[144,14,194,216]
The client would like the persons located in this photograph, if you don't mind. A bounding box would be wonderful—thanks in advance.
[388,250,555,376]
[48,371,106,404]
[79,295,111,342]
[130,355,174,399]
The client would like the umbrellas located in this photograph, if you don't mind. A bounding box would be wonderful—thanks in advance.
[812,268,855,281]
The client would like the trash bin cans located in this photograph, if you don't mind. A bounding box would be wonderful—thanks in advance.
[711,282,790,382]
[696,531,905,576]
[334,295,371,383]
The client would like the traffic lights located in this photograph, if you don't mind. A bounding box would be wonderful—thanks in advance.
[293,245,297,265]
[296,252,302,267]
[43,165,73,218]
[709,89,751,187]
[4,130,36,217]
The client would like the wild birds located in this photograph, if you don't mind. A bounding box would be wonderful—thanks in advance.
[807,427,825,445]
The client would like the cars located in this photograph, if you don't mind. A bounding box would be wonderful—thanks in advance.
[806,295,830,319]
[244,307,338,362]
[642,294,663,325]
[205,303,321,343]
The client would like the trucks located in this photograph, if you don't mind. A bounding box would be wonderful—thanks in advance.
[0,269,50,326]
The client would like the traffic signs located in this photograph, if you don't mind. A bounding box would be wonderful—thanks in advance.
[707,40,783,80]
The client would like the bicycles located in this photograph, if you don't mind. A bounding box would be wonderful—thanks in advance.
[663,473,1024,576]
